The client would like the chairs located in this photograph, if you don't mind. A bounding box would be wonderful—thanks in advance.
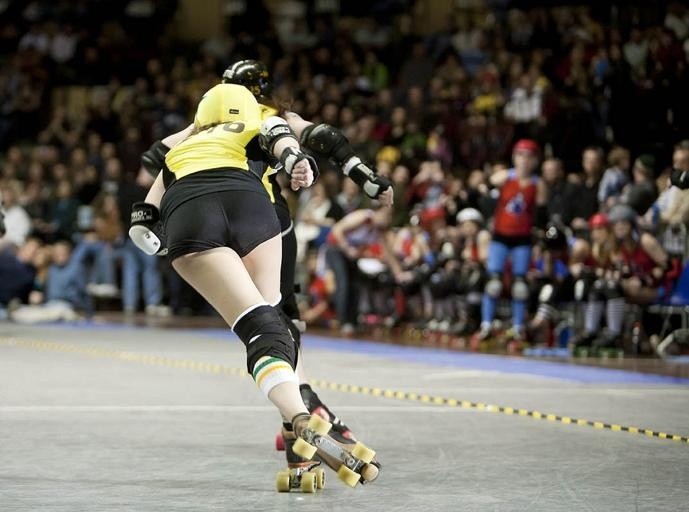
[648,260,689,358]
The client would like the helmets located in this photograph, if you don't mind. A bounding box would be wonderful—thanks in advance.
[193,83,264,130]
[544,225,567,252]
[420,207,446,223]
[222,59,275,100]
[604,205,637,224]
[514,138,543,157]
[455,207,485,227]
[589,213,610,231]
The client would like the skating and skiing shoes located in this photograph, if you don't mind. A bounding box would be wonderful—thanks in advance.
[291,407,381,488]
[368,313,628,361]
[298,384,354,439]
[275,426,327,493]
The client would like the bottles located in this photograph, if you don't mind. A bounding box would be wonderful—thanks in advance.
[630,321,642,356]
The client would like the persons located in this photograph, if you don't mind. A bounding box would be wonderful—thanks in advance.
[134,56,397,452]
[0,1,688,361]
[126,83,383,484]
